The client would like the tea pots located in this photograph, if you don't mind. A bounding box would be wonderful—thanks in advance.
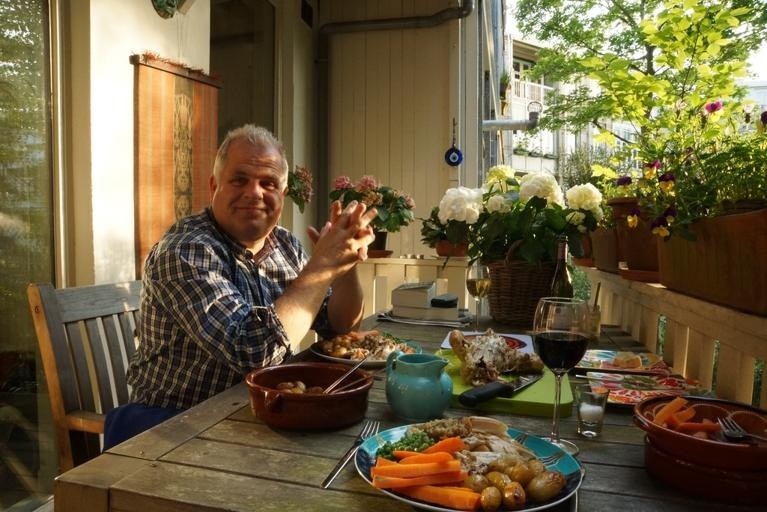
[386,353,455,421]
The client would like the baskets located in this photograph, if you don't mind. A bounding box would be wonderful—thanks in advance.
[488,240,564,326]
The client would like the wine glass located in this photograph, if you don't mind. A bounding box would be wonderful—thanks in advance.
[531,297,590,456]
[466,266,490,332]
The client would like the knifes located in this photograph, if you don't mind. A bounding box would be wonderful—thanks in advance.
[458,372,543,408]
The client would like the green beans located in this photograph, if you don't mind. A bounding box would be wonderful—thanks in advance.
[375,430,435,460]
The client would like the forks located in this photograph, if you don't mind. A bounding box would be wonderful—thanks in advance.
[321,420,380,488]
[716,416,767,442]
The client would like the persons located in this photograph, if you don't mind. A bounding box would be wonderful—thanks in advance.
[126,125,378,411]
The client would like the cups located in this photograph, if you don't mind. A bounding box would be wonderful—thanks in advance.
[575,385,610,438]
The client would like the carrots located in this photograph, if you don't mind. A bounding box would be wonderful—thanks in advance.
[370,435,480,511]
[653,396,722,433]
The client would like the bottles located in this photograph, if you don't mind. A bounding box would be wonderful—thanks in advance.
[550,242,572,303]
[585,305,601,336]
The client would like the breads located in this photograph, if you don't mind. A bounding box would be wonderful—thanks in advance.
[613,351,643,368]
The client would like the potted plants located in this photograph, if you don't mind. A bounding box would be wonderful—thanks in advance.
[589,197,618,273]
[416,206,480,257]
[601,179,659,284]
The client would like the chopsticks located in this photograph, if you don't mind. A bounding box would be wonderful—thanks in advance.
[323,357,386,393]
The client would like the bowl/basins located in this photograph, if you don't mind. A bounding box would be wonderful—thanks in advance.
[245,361,373,430]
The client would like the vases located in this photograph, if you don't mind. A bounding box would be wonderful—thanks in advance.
[367,232,393,257]
[656,206,767,318]
[483,260,573,324]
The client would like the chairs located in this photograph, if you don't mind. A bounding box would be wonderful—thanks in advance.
[27,280,144,473]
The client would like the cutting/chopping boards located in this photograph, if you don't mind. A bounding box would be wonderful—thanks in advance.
[432,348,573,418]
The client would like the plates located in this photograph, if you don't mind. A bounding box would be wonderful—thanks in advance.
[309,340,422,367]
[574,349,663,373]
[586,370,692,406]
[632,395,767,472]
[643,434,766,505]
[353,422,583,512]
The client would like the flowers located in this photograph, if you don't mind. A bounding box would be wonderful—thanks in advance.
[437,163,605,270]
[287,165,315,214]
[614,98,767,241]
[329,174,416,233]
